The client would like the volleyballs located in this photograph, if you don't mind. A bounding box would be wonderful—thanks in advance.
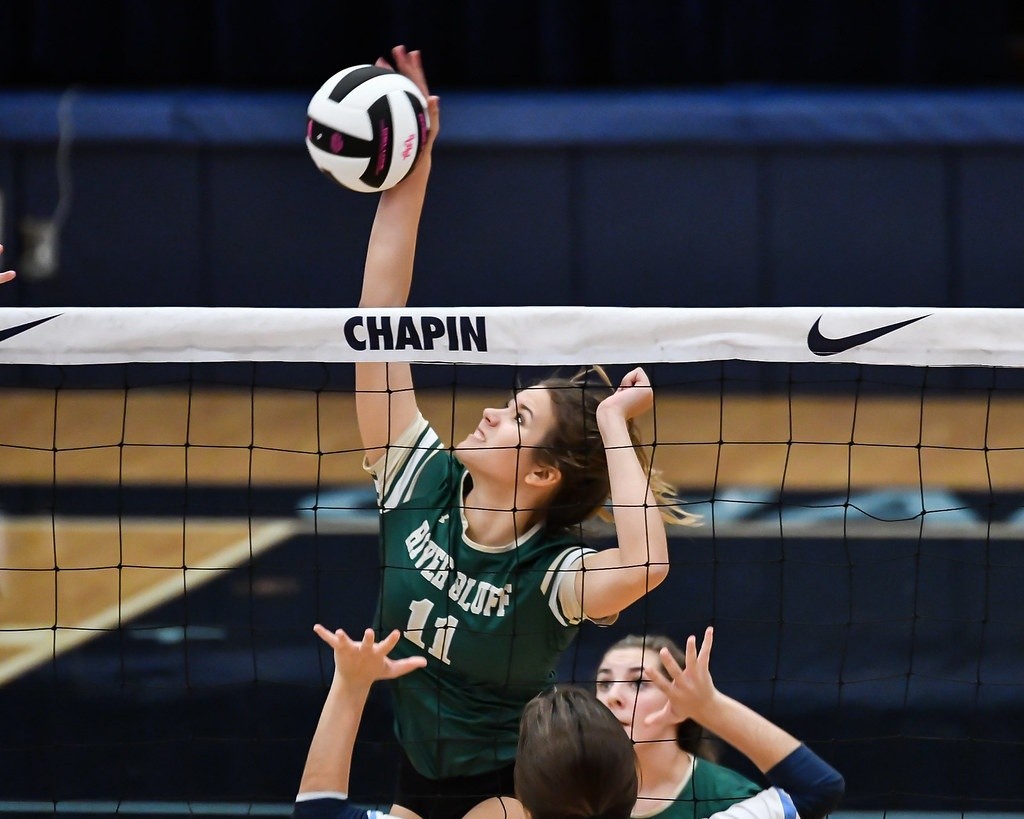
[308,65,429,191]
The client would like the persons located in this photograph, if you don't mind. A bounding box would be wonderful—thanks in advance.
[355,40,699,819]
[288,623,843,818]
[596,631,764,818]
